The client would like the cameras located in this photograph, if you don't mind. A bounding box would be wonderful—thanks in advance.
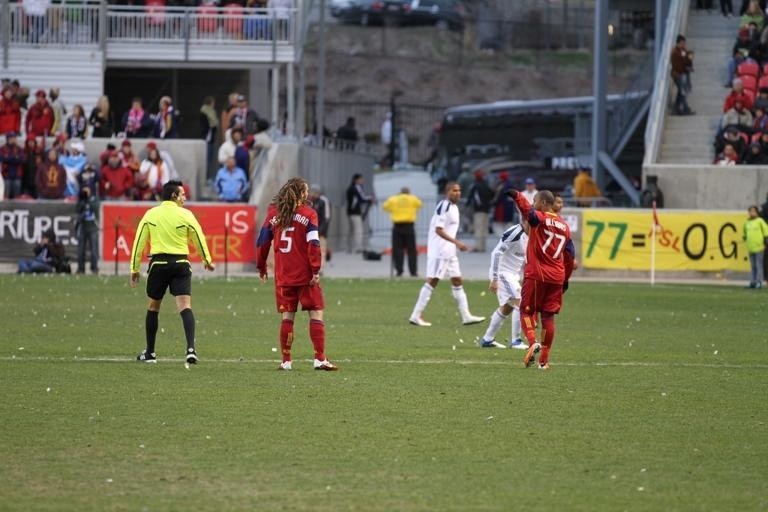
[80,191,87,198]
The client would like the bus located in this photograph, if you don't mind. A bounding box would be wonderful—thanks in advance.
[425,86,650,193]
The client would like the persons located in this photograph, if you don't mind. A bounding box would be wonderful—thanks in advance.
[307,183,332,254]
[383,186,421,279]
[457,163,540,226]
[425,122,442,171]
[670,0,768,165]
[128,181,217,363]
[343,173,373,255]
[574,166,600,207]
[381,112,393,168]
[255,177,337,370]
[312,115,359,150]
[0,77,269,276]
[408,182,486,327]
[743,206,768,290]
[480,187,578,369]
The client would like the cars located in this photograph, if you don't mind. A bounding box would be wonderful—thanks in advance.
[469,169,575,208]
[329,0,468,33]
[452,148,546,209]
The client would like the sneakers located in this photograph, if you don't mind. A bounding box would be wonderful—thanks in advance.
[313,357,338,371]
[137,352,157,363]
[186,347,199,364]
[480,338,506,349]
[462,315,486,325]
[409,317,431,327]
[279,360,292,369]
[510,338,550,370]
